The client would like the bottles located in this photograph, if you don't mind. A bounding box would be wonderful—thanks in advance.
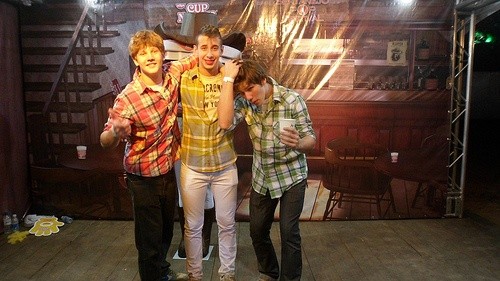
[417,38,430,61]
[3,212,12,234]
[11,214,19,232]
[61,216,72,224]
[425,69,438,90]
[414,66,424,89]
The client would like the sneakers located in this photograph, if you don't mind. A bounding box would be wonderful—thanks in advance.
[167,269,189,281]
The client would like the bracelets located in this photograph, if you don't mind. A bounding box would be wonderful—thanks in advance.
[222,77,234,83]
[110,126,120,139]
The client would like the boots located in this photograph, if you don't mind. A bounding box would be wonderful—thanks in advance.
[176,206,187,258]
[202,207,215,258]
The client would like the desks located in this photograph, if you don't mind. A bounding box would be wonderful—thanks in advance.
[375,148,448,217]
[56,141,126,218]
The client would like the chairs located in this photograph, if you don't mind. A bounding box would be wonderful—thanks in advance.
[321,134,450,220]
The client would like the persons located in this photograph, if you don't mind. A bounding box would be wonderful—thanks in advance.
[218,58,317,281]
[100,30,227,281]
[178,24,239,281]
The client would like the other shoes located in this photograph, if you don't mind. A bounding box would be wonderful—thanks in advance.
[256,273,273,281]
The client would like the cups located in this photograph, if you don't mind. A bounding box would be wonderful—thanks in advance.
[76,145,87,159]
[280,119,296,137]
[391,152,398,163]
